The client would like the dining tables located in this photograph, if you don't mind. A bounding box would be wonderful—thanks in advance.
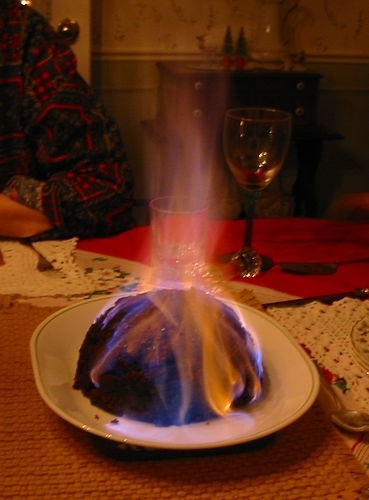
[0,217,369,500]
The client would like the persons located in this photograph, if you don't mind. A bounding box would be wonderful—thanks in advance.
[0,0,137,242]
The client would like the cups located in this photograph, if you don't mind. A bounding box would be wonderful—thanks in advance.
[152,197,211,278]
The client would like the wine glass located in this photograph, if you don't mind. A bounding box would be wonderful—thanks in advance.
[223,108,291,269]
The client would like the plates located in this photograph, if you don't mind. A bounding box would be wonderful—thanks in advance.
[351,315,369,374]
[28,288,319,449]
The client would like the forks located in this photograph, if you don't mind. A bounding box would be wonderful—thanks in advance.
[19,239,67,280]
